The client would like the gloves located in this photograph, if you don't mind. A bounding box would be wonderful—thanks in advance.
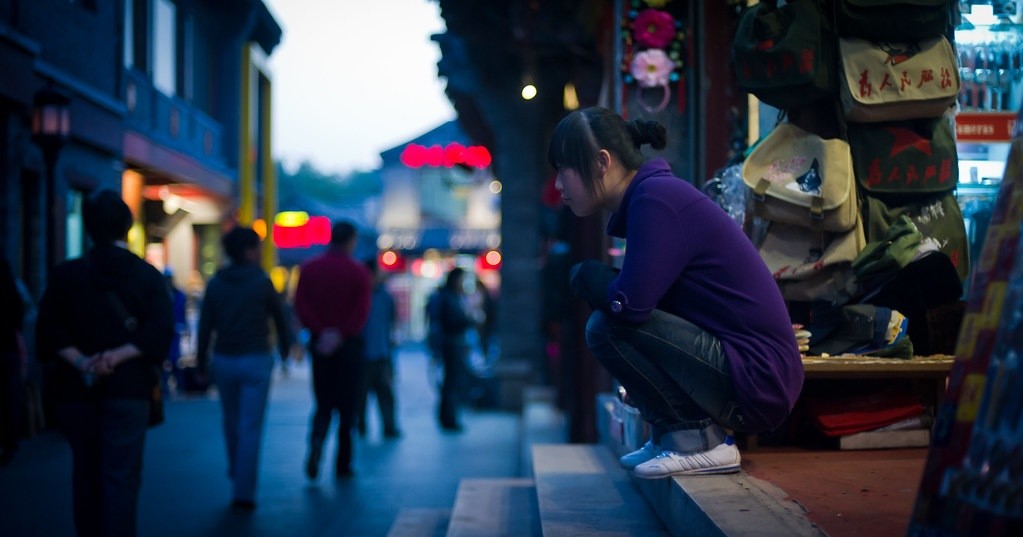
[567,258,617,313]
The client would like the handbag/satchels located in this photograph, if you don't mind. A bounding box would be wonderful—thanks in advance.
[750,211,868,305]
[730,0,837,111]
[742,121,859,235]
[828,0,970,281]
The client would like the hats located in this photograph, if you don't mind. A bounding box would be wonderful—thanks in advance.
[80,190,133,243]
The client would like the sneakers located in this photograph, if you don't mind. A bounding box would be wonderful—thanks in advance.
[617,440,662,470]
[633,435,742,479]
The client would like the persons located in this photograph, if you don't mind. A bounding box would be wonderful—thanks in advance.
[196,228,290,509]
[1,188,186,537]
[295,222,399,481]
[552,107,805,478]
[428,267,475,430]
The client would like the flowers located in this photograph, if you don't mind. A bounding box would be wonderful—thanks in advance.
[630,48,675,89]
[629,8,676,49]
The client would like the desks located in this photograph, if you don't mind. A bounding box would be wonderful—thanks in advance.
[803,356,954,395]
